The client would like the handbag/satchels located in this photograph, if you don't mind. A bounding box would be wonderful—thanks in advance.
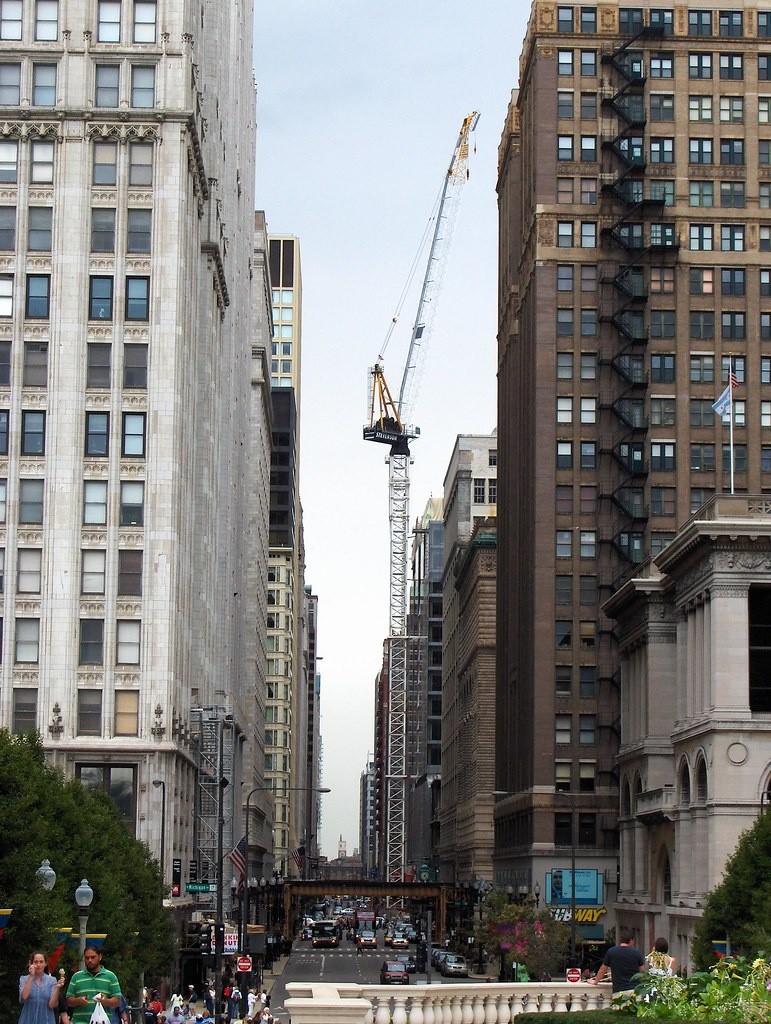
[90,1002,111,1024]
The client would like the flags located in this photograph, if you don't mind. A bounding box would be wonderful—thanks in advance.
[712,386,730,416]
[229,837,245,894]
[291,846,305,870]
[727,364,738,389]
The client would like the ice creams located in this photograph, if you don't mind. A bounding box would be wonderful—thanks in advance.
[59,968,65,978]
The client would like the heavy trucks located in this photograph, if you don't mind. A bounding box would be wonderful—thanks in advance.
[353,909,376,944]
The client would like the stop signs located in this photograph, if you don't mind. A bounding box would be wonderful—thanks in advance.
[565,968,582,982]
[237,956,253,972]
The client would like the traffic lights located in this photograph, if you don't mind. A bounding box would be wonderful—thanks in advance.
[198,925,211,956]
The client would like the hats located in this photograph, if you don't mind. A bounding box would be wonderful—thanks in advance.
[234,986,238,989]
[197,1014,202,1018]
[189,985,194,988]
[264,1007,269,1011]
[247,1017,252,1020]
[248,989,255,994]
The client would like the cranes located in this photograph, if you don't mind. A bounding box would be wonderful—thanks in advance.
[362,107,482,916]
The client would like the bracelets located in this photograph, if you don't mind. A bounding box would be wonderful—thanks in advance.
[124,1020,129,1022]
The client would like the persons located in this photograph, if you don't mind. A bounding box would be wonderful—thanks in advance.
[444,937,451,951]
[337,916,348,930]
[110,992,128,1024]
[356,937,364,956]
[646,936,677,1004]
[18,951,65,1024]
[66,945,121,1024]
[281,936,293,957]
[586,929,646,994]
[338,911,343,915]
[53,991,71,1024]
[323,915,337,920]
[142,961,282,1024]
[346,931,350,941]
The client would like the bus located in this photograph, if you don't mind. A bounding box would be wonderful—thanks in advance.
[310,919,342,949]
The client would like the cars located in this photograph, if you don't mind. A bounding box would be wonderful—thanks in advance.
[358,931,378,949]
[395,953,417,974]
[302,896,386,941]
[379,960,410,985]
[384,928,399,946]
[431,948,470,979]
[390,933,410,949]
[393,919,422,944]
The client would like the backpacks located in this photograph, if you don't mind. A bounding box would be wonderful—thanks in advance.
[192,993,198,1002]
[233,993,239,1003]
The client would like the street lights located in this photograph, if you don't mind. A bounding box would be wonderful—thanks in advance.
[491,791,577,969]
[242,872,285,970]
[239,785,331,1024]
[36,859,56,975]
[153,779,166,908]
[455,879,493,975]
[75,877,95,971]
